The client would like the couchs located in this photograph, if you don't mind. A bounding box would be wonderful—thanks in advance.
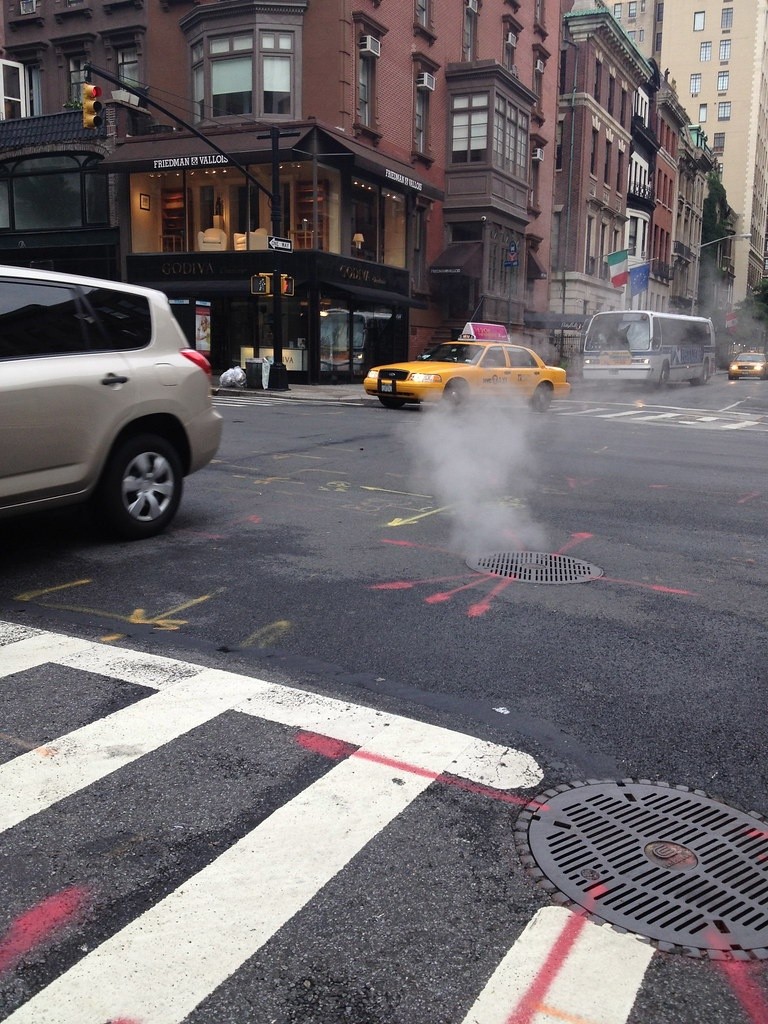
[197,228,227,252]
[233,228,268,250]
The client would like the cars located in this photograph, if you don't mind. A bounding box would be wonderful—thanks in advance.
[362,322,573,414]
[728,349,768,381]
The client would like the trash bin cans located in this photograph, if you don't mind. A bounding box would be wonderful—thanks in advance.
[245,362,262,389]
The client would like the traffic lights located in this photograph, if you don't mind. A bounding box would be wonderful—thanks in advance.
[251,274,269,295]
[281,274,296,296]
[82,82,103,130]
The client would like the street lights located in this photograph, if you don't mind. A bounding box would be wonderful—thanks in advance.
[690,233,752,315]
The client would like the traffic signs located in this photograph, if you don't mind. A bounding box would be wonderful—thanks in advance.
[267,235,293,254]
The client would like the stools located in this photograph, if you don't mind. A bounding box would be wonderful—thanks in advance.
[159,234,184,253]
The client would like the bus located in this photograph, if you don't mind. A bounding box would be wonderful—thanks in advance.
[581,310,717,386]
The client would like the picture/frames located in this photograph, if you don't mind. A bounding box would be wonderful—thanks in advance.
[139,194,151,212]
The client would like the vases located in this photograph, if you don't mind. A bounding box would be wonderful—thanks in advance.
[110,89,130,102]
[129,91,140,106]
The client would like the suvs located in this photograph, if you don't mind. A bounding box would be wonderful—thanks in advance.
[0,264,224,540]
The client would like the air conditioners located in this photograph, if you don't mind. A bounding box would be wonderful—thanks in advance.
[415,72,436,92]
[358,35,381,59]
[531,147,545,163]
[466,0,479,16]
[506,32,517,51]
[18,0,37,16]
[535,59,545,75]
